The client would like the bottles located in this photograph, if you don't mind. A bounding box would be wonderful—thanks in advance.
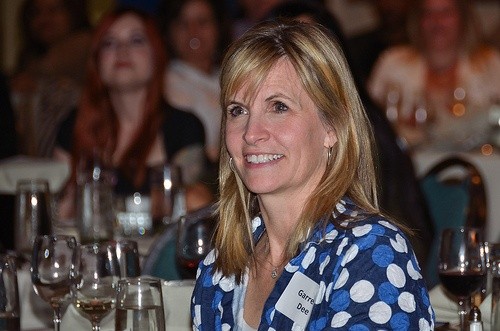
[78,160,186,281]
[466,306,483,331]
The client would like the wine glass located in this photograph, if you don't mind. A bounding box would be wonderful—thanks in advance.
[29,234,120,331]
[438,227,488,331]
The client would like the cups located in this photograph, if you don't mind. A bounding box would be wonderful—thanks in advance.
[178,215,216,280]
[0,252,20,331]
[470,243,500,331]
[14,179,55,260]
[114,277,166,331]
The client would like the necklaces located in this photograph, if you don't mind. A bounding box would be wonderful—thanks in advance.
[265,236,288,278]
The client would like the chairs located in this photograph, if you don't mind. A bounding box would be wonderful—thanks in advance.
[418,157,487,235]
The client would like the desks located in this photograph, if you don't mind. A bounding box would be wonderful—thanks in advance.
[57,285,492,331]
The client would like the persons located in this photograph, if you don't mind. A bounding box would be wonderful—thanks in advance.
[0,0,500,290]
[190,19,435,331]
